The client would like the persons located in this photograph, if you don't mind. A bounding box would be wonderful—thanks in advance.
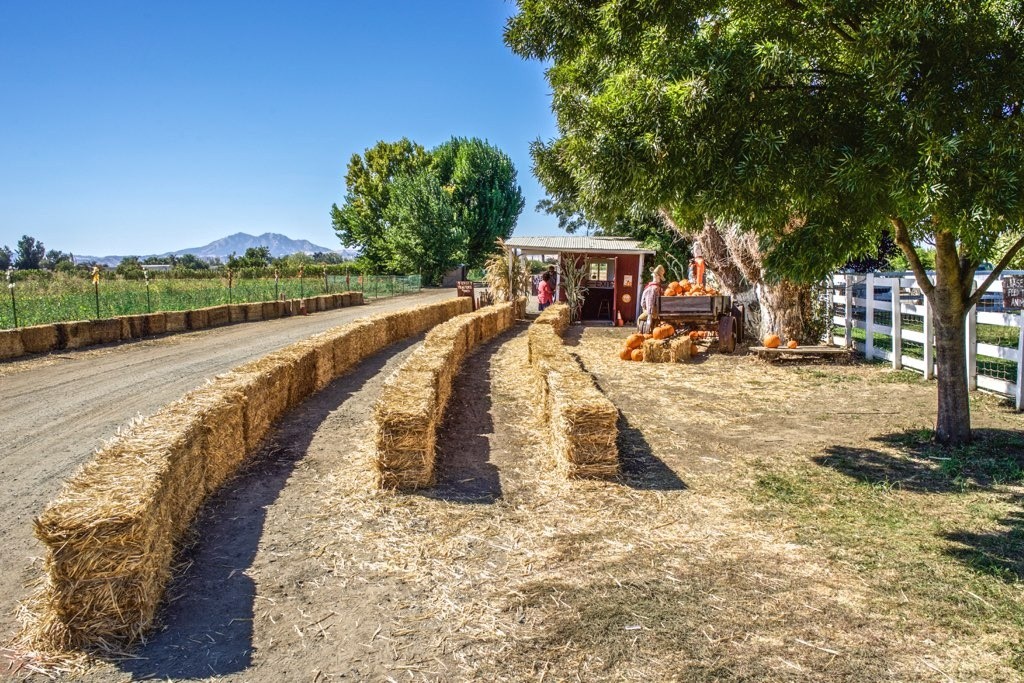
[537,266,557,312]
[638,265,666,333]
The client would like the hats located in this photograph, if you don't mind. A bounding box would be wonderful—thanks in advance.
[651,265,666,281]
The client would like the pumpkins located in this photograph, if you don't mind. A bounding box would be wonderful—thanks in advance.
[661,278,718,296]
[764,333,781,348]
[787,338,797,348]
[619,323,710,361]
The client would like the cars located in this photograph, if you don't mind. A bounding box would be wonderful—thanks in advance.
[973,262,1002,304]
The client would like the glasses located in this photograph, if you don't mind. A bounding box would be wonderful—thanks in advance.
[549,270,554,272]
